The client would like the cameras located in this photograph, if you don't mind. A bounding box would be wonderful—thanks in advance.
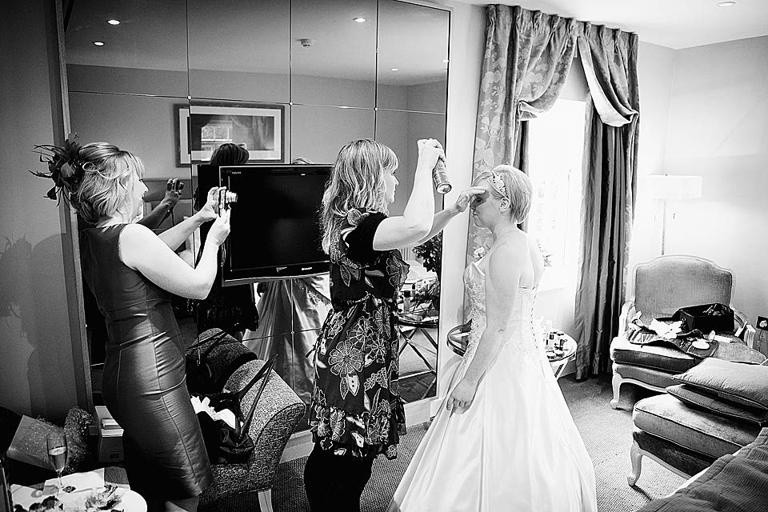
[218,190,238,218]
[167,178,185,193]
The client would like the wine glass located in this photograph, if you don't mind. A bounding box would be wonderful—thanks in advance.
[46,432,68,490]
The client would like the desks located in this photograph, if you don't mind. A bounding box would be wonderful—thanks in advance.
[392,293,437,406]
[446,322,578,385]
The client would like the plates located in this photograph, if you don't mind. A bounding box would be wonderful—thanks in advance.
[57,487,148,512]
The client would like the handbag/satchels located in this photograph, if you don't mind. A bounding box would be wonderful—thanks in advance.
[188,352,280,464]
[186,320,240,381]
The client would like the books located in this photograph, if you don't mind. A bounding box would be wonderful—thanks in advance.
[94,406,124,437]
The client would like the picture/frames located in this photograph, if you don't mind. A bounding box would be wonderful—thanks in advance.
[169,100,290,172]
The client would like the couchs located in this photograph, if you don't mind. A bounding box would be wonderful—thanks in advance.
[182,328,256,400]
[627,357,766,512]
[609,254,756,413]
[189,359,307,512]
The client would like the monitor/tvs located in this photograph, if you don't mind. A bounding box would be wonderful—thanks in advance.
[220,166,335,288]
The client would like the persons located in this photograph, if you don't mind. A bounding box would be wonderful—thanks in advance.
[382,163,597,512]
[31,135,230,512]
[244,156,334,404]
[303,139,489,512]
[195,143,258,343]
[138,178,183,230]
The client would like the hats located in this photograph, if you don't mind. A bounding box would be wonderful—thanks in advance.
[211,143,249,165]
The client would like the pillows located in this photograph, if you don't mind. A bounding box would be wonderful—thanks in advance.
[665,386,765,433]
[673,359,766,412]
